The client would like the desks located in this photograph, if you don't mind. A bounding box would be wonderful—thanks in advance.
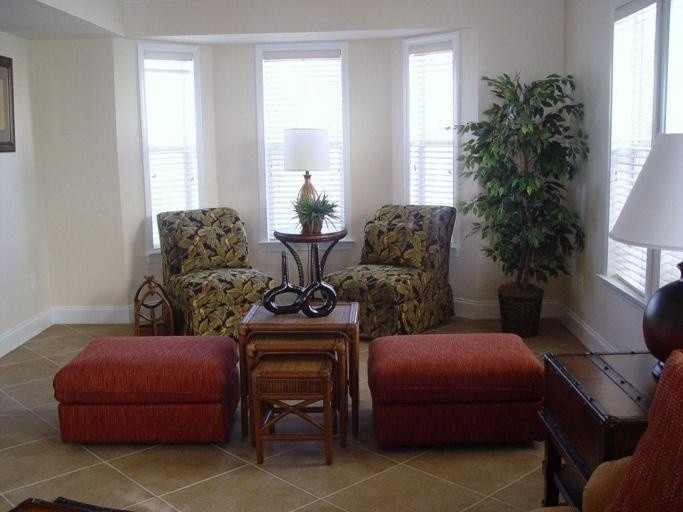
[533,352,662,512]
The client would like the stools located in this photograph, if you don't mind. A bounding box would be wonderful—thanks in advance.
[251,356,335,463]
[365,332,547,446]
[247,338,349,447]
[53,334,241,445]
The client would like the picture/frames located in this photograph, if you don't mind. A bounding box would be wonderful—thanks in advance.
[0,54,17,152]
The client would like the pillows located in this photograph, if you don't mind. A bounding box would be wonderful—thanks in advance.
[359,218,431,270]
[171,221,252,275]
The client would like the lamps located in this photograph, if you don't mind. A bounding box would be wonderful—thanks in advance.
[607,133,682,380]
[283,128,331,200]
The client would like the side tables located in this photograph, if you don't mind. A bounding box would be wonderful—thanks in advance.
[238,301,361,441]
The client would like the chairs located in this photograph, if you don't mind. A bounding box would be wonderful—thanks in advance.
[155,207,278,335]
[322,203,457,340]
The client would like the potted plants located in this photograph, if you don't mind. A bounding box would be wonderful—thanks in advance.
[289,194,344,236]
[456,73,593,339]
